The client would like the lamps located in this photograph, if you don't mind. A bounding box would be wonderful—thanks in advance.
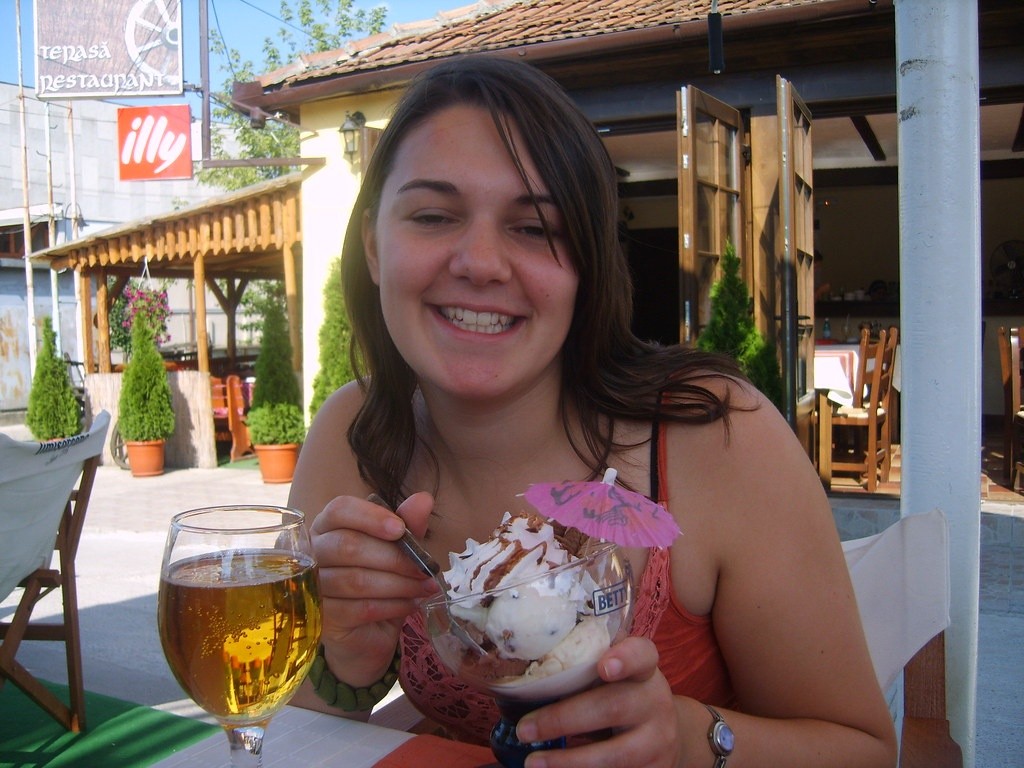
[337,110,368,164]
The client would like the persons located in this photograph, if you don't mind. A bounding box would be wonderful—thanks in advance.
[274,56,897,768]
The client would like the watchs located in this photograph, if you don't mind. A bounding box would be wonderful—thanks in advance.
[703,703,735,768]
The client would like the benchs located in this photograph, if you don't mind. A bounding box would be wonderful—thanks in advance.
[210,375,256,462]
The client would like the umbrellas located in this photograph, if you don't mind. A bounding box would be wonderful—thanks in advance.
[522,468,684,581]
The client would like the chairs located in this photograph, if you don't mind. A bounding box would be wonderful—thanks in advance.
[845,511,956,768]
[0,409,111,733]
[819,327,899,493]
[996,326,1024,489]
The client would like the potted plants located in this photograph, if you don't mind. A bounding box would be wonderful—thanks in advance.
[246,302,304,484]
[119,308,176,478]
[25,316,84,445]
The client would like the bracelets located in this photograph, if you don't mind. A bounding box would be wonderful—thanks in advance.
[309,641,402,711]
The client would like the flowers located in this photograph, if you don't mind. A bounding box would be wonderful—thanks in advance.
[113,285,172,343]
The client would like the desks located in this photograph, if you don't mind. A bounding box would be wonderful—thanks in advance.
[813,344,901,418]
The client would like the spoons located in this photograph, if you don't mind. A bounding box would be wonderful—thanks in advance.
[367,493,488,654]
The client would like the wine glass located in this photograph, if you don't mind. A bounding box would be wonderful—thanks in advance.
[420,540,635,768]
[156,504,323,768]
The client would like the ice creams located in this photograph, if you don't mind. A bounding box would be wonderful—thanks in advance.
[441,508,620,694]
